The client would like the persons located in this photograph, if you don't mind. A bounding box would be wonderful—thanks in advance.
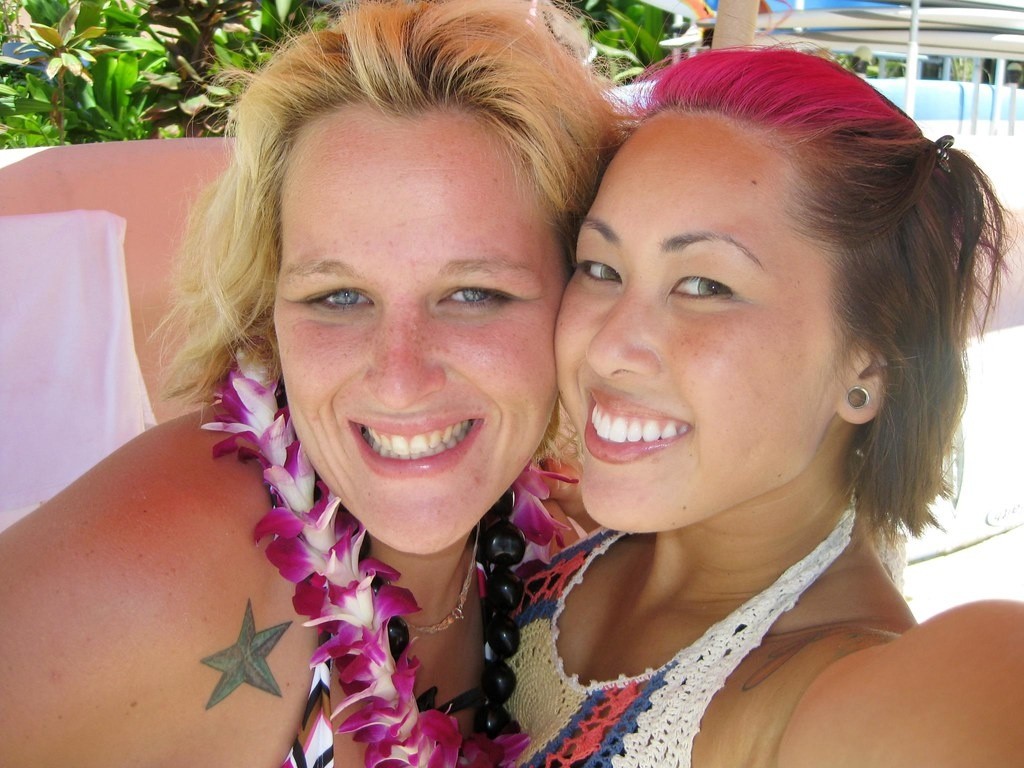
[4,1,676,767]
[475,43,1024,768]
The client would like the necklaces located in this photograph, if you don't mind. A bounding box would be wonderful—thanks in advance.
[195,344,581,767]
